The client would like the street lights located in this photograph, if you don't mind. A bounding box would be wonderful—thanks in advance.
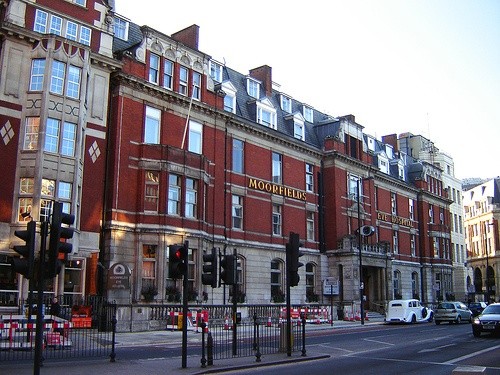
[356,176,374,324]
[484,222,496,303]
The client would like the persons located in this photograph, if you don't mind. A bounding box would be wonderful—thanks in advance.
[49,297,61,316]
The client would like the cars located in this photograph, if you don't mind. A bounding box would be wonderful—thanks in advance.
[433,301,474,325]
[468,301,488,317]
[384,297,434,325]
[471,302,500,338]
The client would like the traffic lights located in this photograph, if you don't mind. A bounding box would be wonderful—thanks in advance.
[201,248,217,288]
[48,202,76,259]
[220,254,235,285]
[12,220,37,279]
[168,244,187,279]
[289,231,304,272]
